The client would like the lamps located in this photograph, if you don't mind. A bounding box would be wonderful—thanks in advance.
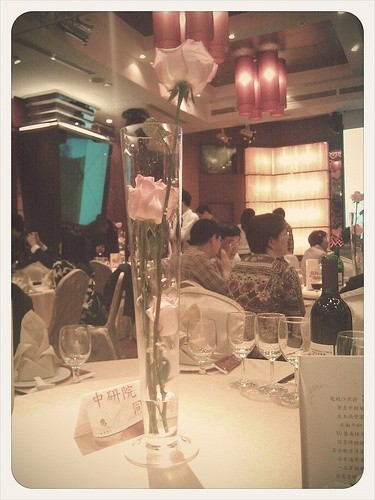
[234,47,289,121]
[152,11,229,64]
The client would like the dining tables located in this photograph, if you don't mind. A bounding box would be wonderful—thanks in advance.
[11,357,303,490]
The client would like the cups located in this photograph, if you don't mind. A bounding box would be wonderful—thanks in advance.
[334,330,364,356]
[295,269,304,290]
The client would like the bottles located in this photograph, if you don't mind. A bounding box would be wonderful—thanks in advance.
[334,246,344,287]
[310,253,353,356]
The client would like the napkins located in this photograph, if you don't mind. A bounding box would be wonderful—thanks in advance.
[12,310,61,383]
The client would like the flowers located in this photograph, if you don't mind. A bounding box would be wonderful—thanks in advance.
[125,40,220,430]
[349,192,364,274]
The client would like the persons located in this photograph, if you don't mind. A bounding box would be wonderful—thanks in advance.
[211,221,241,277]
[236,208,255,260]
[166,218,232,296]
[11,214,119,357]
[227,214,306,362]
[197,205,218,224]
[339,227,361,260]
[166,189,199,257]
[301,230,329,286]
[273,208,294,254]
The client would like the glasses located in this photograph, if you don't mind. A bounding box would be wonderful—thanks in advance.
[221,241,241,247]
[276,232,290,240]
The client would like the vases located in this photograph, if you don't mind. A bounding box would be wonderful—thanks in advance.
[348,221,364,280]
[120,122,200,467]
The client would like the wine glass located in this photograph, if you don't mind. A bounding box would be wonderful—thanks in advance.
[187,317,217,376]
[58,324,92,385]
[227,310,259,390]
[254,312,288,398]
[277,316,311,408]
[311,274,322,300]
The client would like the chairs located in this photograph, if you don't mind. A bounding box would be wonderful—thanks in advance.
[46,268,89,358]
[82,272,124,361]
[178,289,243,356]
[90,260,116,296]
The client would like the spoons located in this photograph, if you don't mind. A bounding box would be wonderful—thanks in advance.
[33,376,55,391]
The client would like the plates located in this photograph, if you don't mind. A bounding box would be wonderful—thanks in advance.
[179,362,215,371]
[15,366,71,389]
